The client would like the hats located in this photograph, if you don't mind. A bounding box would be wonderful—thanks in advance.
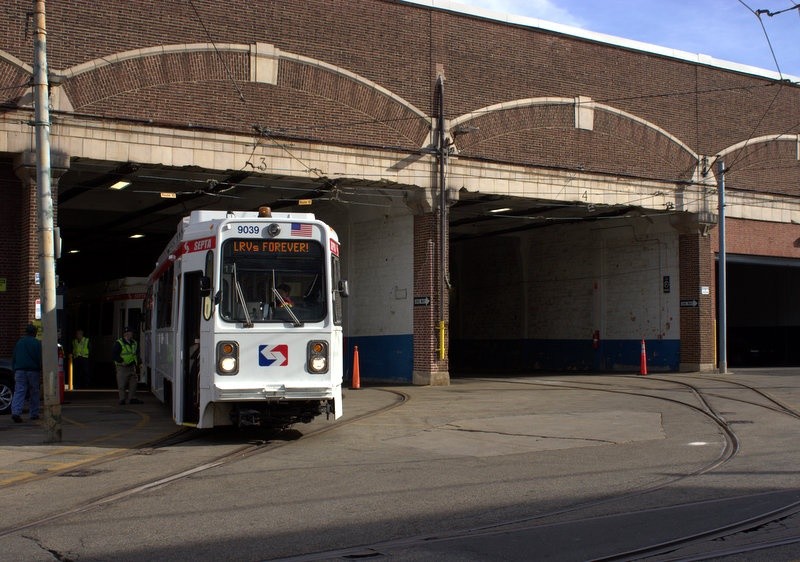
[122,327,133,334]
[26,324,37,337]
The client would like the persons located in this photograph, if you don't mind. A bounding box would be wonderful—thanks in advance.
[70,329,91,390]
[275,284,291,306]
[112,327,146,404]
[9,323,42,422]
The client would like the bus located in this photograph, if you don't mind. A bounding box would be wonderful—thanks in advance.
[103,206,350,434]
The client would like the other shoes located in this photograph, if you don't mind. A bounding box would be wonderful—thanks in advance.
[119,400,125,405]
[129,399,144,405]
[12,414,24,424]
[31,416,39,420]
